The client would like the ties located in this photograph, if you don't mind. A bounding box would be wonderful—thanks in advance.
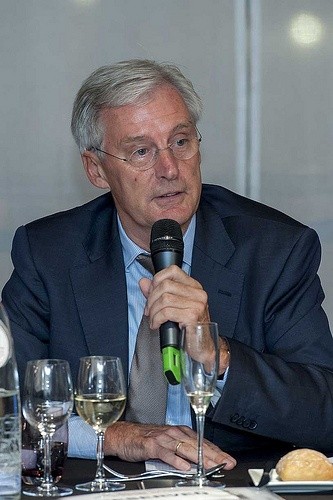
[124,251,169,426]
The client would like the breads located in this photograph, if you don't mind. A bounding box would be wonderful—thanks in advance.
[275,448,333,481]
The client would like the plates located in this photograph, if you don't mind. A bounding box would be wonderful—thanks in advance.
[268,469,333,493]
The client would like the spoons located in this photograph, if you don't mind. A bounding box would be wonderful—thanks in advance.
[103,461,228,482]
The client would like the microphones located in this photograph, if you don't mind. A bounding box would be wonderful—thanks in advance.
[150,218,185,385]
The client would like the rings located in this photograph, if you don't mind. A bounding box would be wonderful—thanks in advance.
[175,442,183,455]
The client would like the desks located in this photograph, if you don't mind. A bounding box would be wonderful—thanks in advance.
[20,457,333,500]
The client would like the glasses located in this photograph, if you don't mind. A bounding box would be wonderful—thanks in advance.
[87,122,202,172]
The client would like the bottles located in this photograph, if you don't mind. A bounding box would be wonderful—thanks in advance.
[0,294,22,500]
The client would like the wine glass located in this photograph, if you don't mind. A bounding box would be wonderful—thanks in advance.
[73,355,127,490]
[173,320,225,490]
[22,358,74,497]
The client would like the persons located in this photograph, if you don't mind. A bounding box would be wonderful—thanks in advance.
[0,58,333,472]
